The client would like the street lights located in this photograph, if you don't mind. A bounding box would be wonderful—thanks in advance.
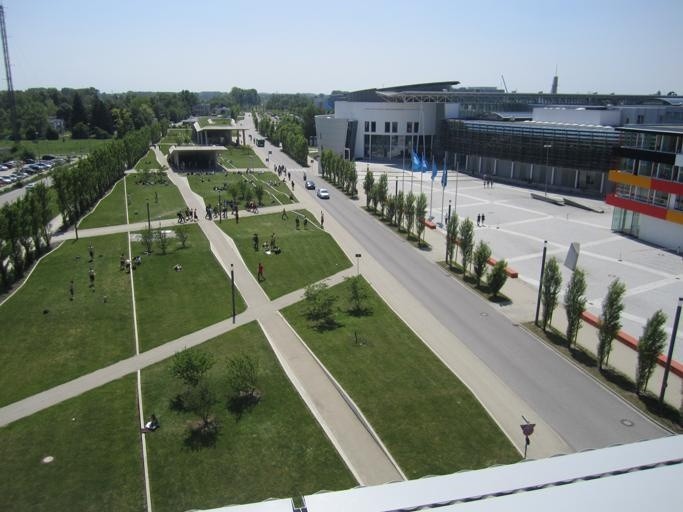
[518,421,535,462]
[146,197,151,230]
[543,144,552,197]
[534,239,550,326]
[229,263,236,324]
[366,163,371,173]
[395,175,399,225]
[445,199,452,264]
[354,253,361,275]
[657,297,683,409]
[218,190,222,224]
[344,147,351,161]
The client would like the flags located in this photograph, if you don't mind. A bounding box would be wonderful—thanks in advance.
[421,155,427,173]
[441,159,447,186]
[431,158,437,179]
[412,152,420,171]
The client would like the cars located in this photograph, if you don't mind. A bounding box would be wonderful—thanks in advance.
[305,180,315,190]
[0,154,70,189]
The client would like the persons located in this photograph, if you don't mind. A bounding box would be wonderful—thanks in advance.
[477,213,481,226]
[69,279,74,299]
[89,268,96,289]
[445,213,448,224]
[258,263,265,281]
[148,414,159,431]
[482,214,485,225]
[177,165,324,253]
[120,252,141,272]
[88,245,93,262]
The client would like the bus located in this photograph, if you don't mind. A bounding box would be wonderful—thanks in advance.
[252,132,265,147]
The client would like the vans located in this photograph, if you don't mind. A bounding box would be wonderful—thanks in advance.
[317,188,330,199]
